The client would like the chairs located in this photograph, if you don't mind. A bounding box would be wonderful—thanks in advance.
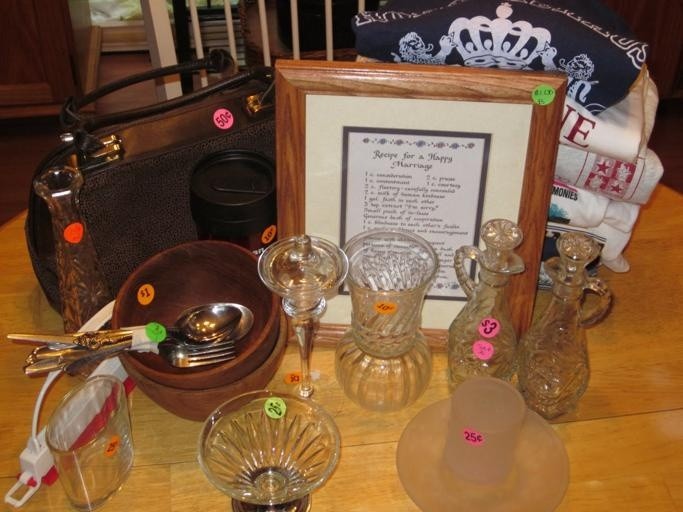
[141,0,366,101]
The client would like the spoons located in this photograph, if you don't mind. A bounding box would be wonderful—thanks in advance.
[6,299,255,379]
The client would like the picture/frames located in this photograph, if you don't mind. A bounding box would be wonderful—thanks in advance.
[273,60,568,354]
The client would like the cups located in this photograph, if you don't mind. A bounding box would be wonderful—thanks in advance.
[43,374,136,510]
[444,374,526,486]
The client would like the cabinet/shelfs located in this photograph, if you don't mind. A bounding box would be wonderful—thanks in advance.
[0,0,103,118]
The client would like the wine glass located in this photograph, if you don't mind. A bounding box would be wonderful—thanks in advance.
[258,233,345,420]
[197,389,340,512]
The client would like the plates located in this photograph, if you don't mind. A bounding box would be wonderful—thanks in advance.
[395,398,570,512]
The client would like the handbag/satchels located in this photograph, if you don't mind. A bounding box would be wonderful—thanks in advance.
[29,49,276,328]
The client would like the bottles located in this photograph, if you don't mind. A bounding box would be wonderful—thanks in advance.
[448,219,525,400]
[339,224,440,412]
[188,147,276,253]
[517,229,613,425]
[31,164,116,335]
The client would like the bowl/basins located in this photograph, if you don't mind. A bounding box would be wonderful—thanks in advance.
[111,239,281,391]
[108,305,290,425]
